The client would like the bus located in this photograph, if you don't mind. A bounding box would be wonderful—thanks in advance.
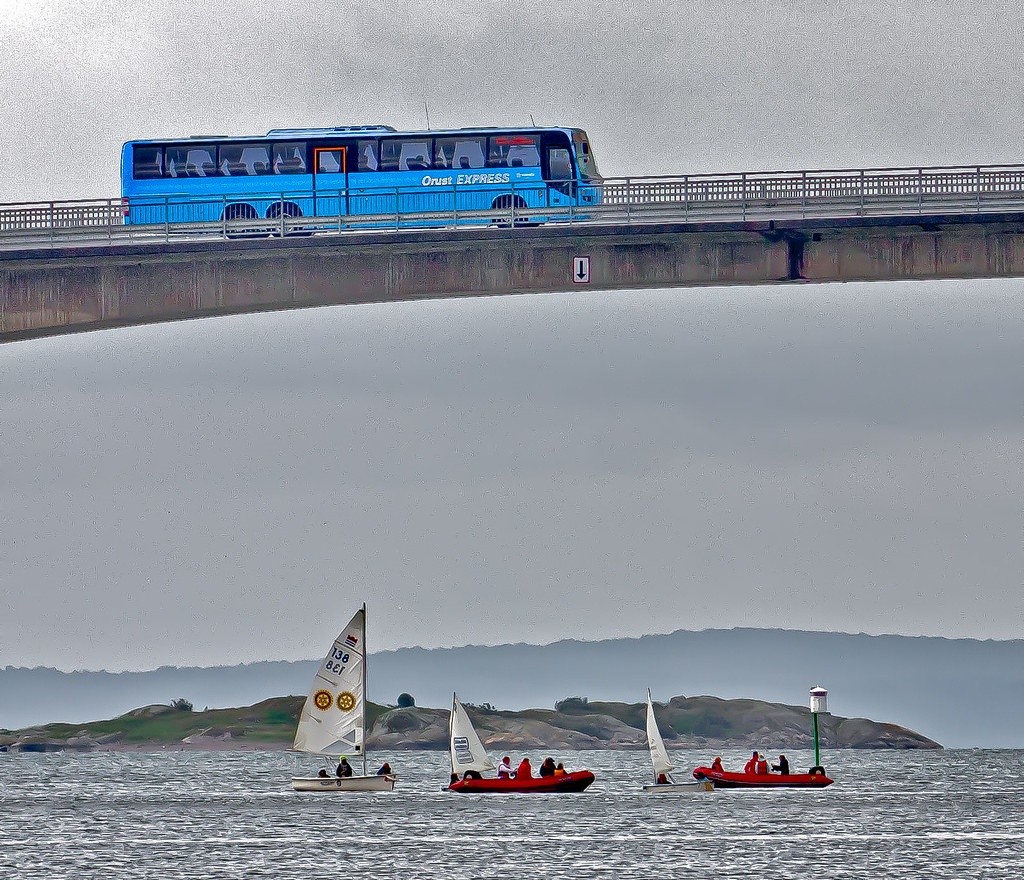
[119,124,604,239]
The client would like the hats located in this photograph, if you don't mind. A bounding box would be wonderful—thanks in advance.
[503,757,510,763]
[340,756,348,760]
[780,755,785,759]
[547,758,554,762]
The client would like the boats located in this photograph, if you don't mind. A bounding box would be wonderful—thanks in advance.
[693,685,835,788]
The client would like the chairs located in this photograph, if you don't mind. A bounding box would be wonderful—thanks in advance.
[174,162,305,177]
[460,157,471,168]
[406,158,417,170]
[512,158,523,167]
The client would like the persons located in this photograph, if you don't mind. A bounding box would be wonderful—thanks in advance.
[362,155,375,172]
[437,157,445,167]
[658,774,671,784]
[517,758,531,779]
[772,755,789,775]
[337,757,352,777]
[540,757,567,777]
[498,757,517,779]
[318,770,331,778]
[745,751,770,774]
[284,157,304,172]
[376,763,391,775]
[416,154,431,169]
[712,758,724,772]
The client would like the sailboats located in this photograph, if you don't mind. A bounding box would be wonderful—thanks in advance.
[290,600,399,792]
[639,687,718,791]
[440,691,596,794]
